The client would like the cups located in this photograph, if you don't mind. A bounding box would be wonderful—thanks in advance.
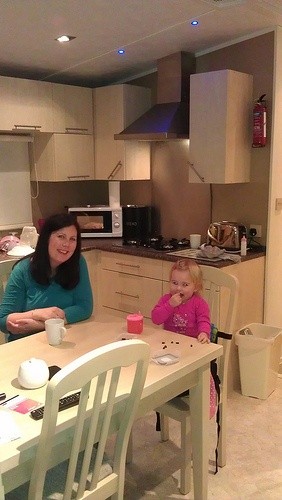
[127,313,144,336]
[190,234,201,249]
[44,318,68,345]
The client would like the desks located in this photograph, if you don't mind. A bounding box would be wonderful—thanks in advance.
[0,314,224,500]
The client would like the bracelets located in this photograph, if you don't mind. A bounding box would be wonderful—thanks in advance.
[32,309,36,320]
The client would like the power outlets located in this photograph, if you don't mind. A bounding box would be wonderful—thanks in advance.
[249,225,261,237]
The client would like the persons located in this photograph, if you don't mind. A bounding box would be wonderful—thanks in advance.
[0,213,92,344]
[152,259,217,420]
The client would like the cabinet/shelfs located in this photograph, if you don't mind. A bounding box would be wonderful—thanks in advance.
[0,75,150,181]
[186,69,254,184]
[0,250,264,401]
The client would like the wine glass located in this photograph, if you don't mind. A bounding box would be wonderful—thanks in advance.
[7,232,18,251]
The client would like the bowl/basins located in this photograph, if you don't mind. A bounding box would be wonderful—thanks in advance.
[6,245,35,255]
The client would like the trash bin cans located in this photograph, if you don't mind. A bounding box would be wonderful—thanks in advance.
[236,323,281,400]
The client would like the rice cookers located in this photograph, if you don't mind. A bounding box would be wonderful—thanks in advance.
[208,220,242,249]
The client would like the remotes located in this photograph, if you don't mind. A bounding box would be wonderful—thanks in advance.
[30,391,81,421]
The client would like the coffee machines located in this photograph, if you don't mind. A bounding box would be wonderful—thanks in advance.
[123,205,151,244]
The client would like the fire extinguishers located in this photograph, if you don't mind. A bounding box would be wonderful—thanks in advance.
[252,94,268,148]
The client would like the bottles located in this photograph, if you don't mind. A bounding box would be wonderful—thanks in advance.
[240,234,247,256]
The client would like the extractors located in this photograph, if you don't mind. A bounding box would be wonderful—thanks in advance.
[113,51,196,140]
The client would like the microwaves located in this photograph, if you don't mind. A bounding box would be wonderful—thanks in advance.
[64,205,124,238]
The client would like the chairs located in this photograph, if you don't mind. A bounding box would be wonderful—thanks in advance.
[5,339,151,500]
[126,265,238,495]
